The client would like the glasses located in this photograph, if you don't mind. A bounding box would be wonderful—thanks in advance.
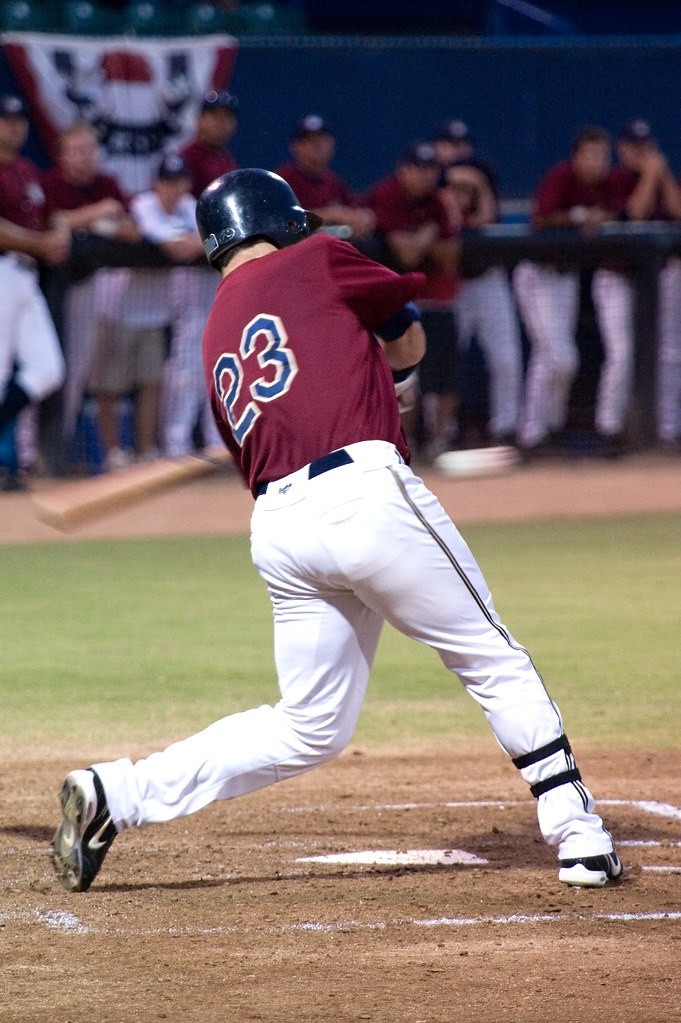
[64,142,96,160]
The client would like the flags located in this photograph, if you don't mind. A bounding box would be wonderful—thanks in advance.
[2,32,240,196]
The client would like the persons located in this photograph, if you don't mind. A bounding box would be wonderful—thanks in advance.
[50,166,623,893]
[0,87,680,498]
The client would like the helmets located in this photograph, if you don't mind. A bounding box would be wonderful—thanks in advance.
[291,114,337,137]
[201,90,242,116]
[405,139,439,166]
[623,117,651,143]
[0,92,29,119]
[440,119,477,145]
[195,167,324,271]
[158,154,186,178]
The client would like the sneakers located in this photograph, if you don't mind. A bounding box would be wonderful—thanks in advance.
[51,768,118,892]
[558,849,623,888]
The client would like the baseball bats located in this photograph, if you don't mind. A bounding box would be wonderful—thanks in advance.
[31,388,415,534]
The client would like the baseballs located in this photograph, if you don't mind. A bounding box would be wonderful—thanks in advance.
[431,445,528,481]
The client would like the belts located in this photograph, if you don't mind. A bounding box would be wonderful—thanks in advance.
[256,449,353,495]
